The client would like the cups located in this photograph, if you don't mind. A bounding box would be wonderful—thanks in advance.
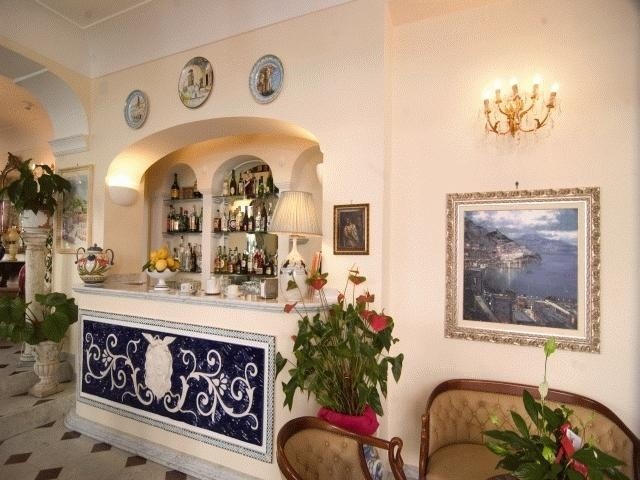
[181,282,193,292]
[225,283,239,293]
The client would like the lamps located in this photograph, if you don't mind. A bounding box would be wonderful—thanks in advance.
[107,185,137,207]
[267,190,323,275]
[482,71,560,140]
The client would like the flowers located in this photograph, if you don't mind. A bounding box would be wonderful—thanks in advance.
[275,257,405,416]
[481,336,629,480]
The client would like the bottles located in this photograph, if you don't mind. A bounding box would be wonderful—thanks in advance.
[214,238,277,276]
[173,232,201,272]
[169,173,181,199]
[167,203,204,231]
[194,179,200,197]
[213,202,272,232]
[222,168,274,196]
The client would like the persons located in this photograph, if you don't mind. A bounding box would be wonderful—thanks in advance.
[343,218,361,248]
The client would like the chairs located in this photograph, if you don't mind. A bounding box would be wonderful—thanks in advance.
[276,416,408,479]
[418,378,640,480]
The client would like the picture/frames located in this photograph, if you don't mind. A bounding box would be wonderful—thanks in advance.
[444,187,602,357]
[55,164,94,255]
[333,202,370,257]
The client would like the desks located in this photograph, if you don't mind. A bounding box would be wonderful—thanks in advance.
[0,253,25,288]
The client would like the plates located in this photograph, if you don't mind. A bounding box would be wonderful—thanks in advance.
[248,54,284,105]
[178,290,196,294]
[223,293,242,298]
[124,88,149,130]
[177,56,215,109]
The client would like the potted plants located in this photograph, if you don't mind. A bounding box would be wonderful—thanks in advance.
[0,157,72,233]
[0,293,77,399]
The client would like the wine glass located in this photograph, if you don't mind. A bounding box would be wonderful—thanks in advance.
[239,280,259,296]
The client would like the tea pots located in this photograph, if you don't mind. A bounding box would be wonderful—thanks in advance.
[207,276,220,293]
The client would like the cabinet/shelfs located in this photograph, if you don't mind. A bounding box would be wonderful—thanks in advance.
[212,191,280,298]
[162,196,203,273]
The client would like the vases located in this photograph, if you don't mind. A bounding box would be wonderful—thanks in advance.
[6,225,21,262]
[316,404,385,480]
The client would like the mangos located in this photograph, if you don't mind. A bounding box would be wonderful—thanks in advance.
[148,247,181,271]
